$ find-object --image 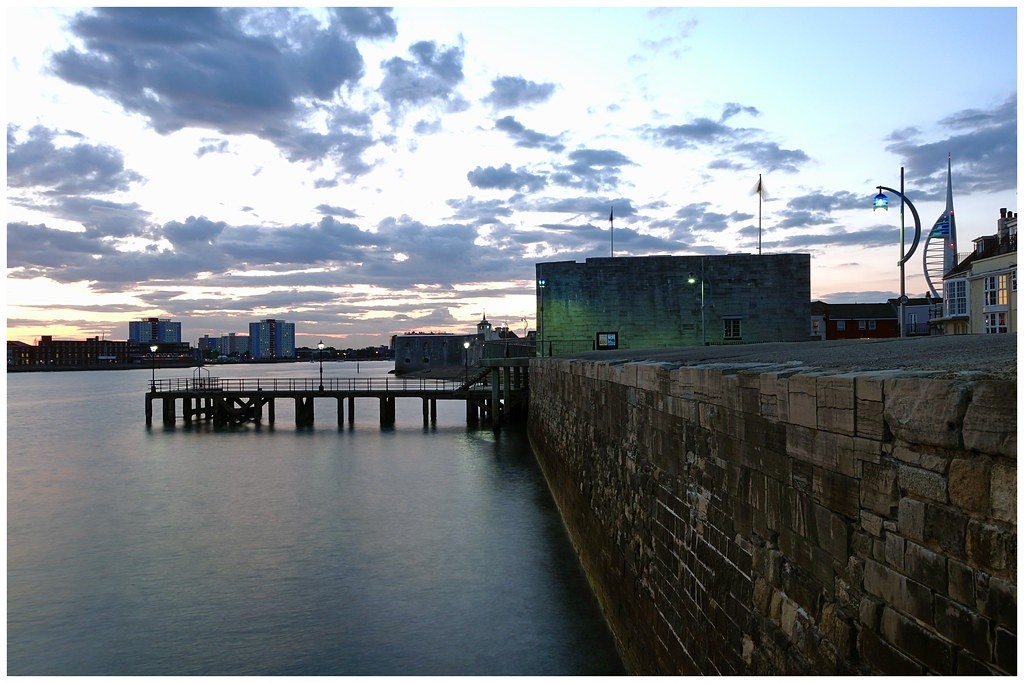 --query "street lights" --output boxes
[689,272,706,345]
[873,167,921,297]
[537,277,546,358]
[462,342,470,383]
[317,340,326,390]
[150,344,158,391]
